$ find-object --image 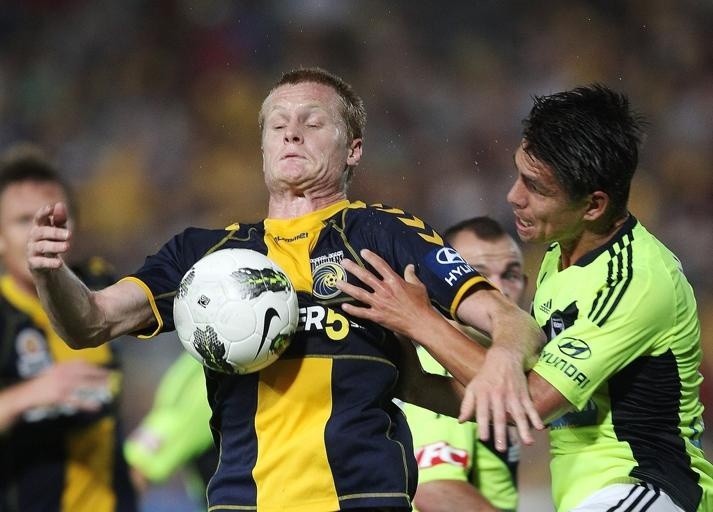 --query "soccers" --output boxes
[173,247,299,375]
